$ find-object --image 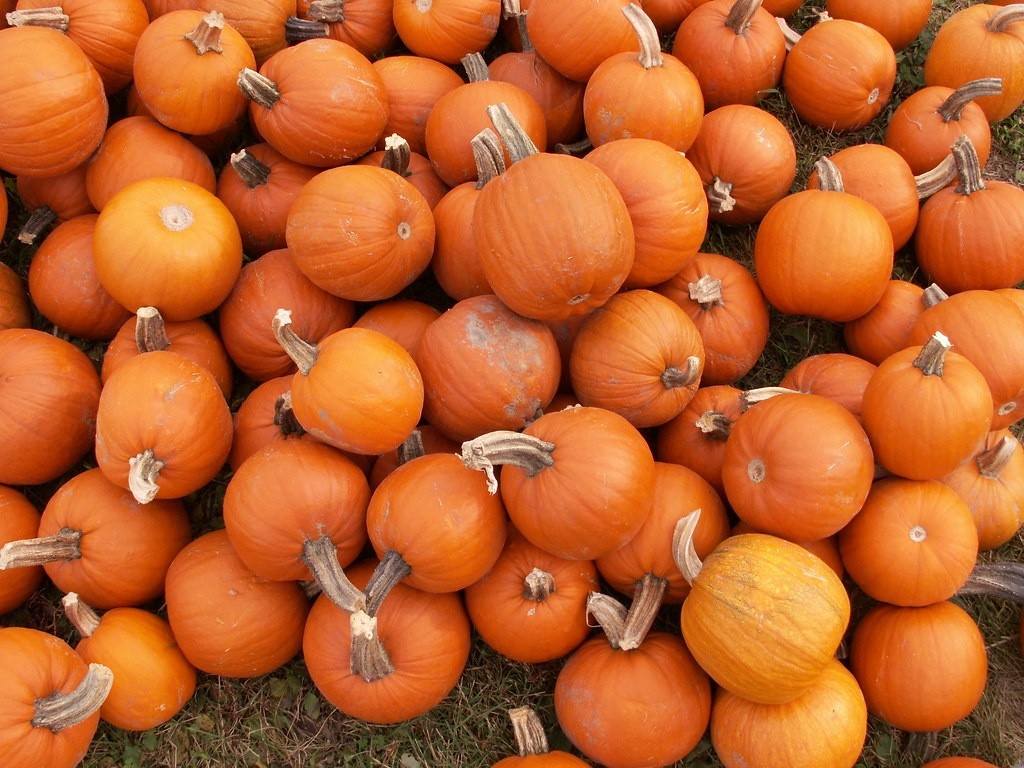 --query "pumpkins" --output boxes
[0,0,1024,768]
